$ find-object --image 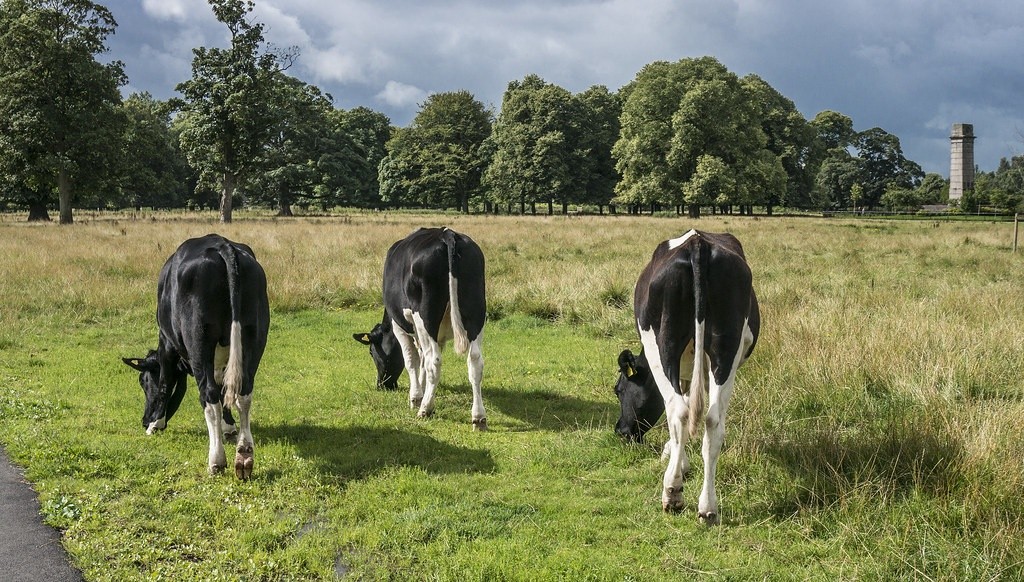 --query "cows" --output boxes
[352,227,486,431]
[121,233,270,482]
[613,228,760,529]
